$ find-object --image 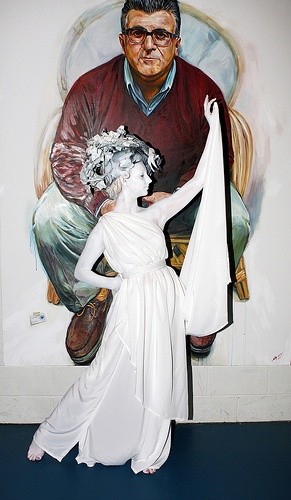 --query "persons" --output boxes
[27,94,220,476]
